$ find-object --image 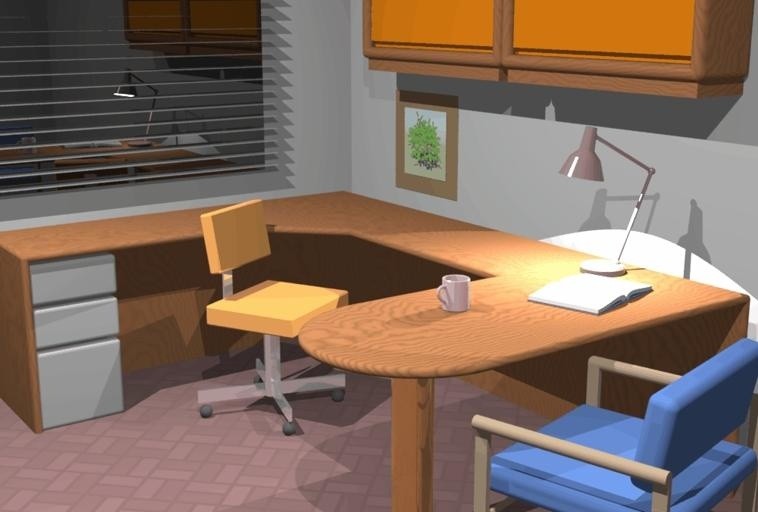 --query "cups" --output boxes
[437,273,471,314]
[15,137,38,155]
